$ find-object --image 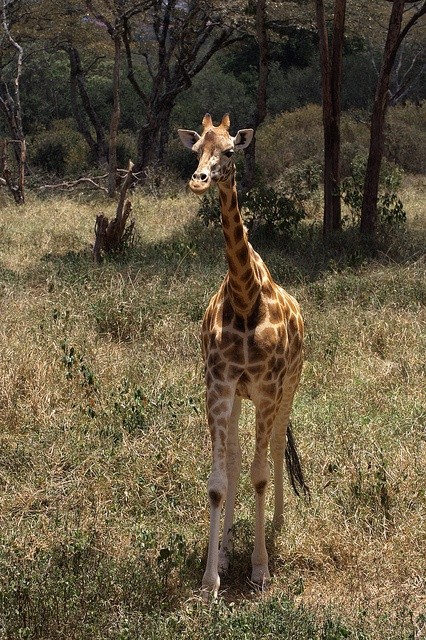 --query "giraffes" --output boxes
[177,112,311,607]
